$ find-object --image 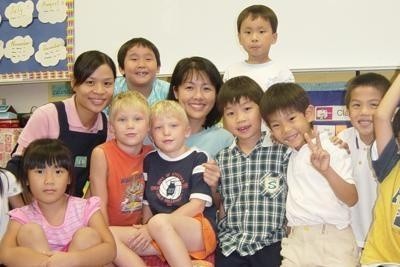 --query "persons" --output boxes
[330,72,400,267]
[222,4,294,92]
[0,37,360,266]
